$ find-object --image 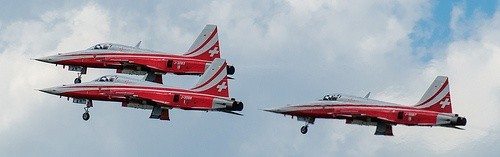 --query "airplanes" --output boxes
[31,24,236,84]
[34,58,245,121]
[260,75,467,137]
